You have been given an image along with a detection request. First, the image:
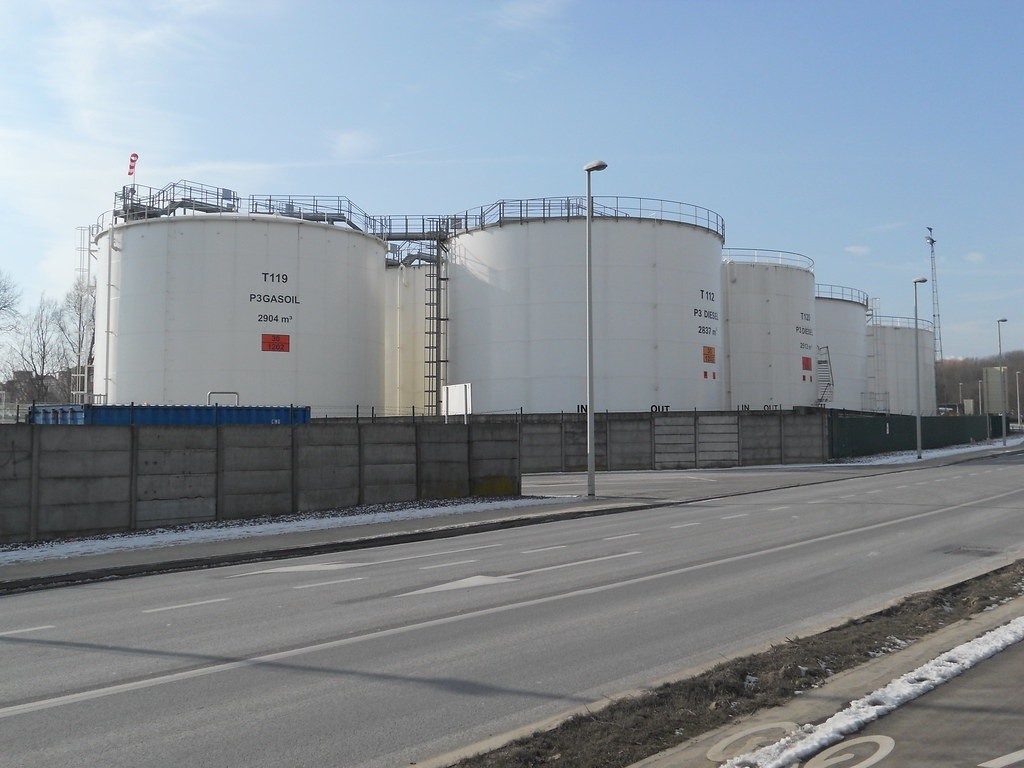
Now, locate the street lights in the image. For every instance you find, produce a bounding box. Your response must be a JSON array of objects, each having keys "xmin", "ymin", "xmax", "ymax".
[
  {"xmin": 979, "ymin": 380, "xmax": 982, "ymax": 416},
  {"xmin": 584, "ymin": 160, "xmax": 608, "ymax": 496},
  {"xmin": 1016, "ymin": 372, "xmax": 1021, "ymax": 425},
  {"xmin": 913, "ymin": 277, "xmax": 927, "ymax": 460},
  {"xmin": 997, "ymin": 318, "xmax": 1007, "ymax": 445},
  {"xmin": 959, "ymin": 383, "xmax": 963, "ymax": 404}
]
